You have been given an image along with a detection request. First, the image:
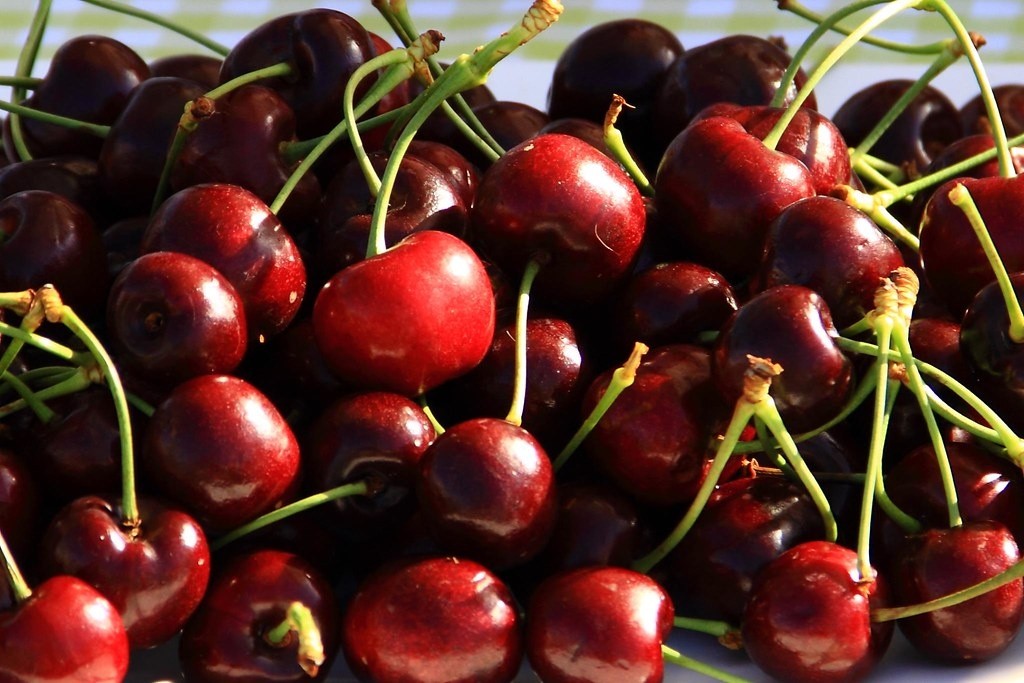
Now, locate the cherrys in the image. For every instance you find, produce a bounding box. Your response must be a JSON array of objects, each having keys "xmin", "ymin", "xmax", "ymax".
[{"xmin": 0, "ymin": 2, "xmax": 1024, "ymax": 683}]
